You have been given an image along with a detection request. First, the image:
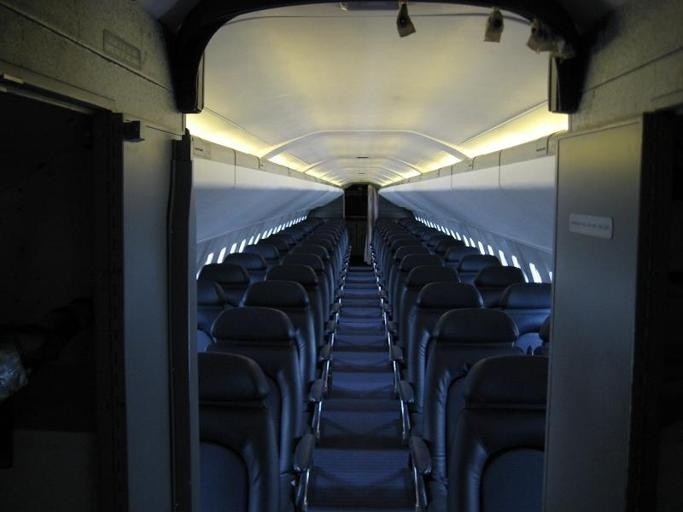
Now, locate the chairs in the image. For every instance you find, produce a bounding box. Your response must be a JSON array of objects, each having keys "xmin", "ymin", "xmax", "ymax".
[
  {"xmin": 194, "ymin": 215, "xmax": 348, "ymax": 512},
  {"xmin": 372, "ymin": 216, "xmax": 552, "ymax": 512}
]
